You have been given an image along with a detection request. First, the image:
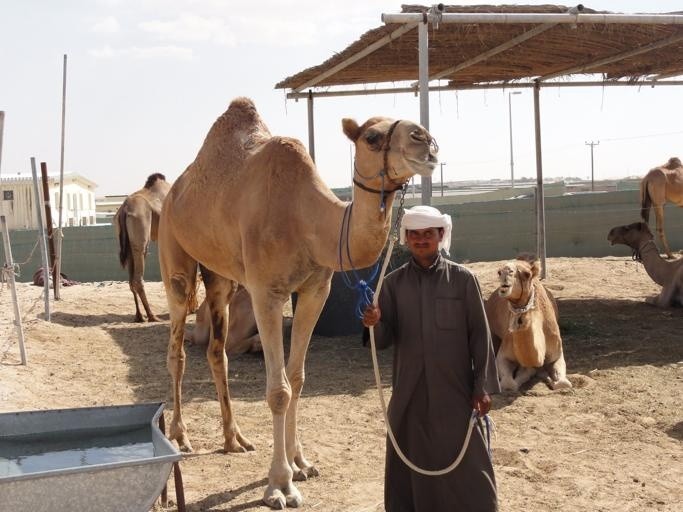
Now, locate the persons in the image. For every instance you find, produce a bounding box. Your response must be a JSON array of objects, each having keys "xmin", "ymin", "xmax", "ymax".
[{"xmin": 360, "ymin": 204, "xmax": 502, "ymax": 511}]
